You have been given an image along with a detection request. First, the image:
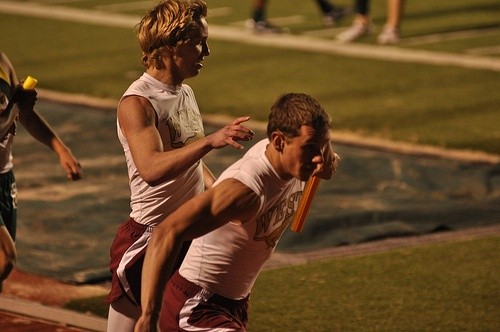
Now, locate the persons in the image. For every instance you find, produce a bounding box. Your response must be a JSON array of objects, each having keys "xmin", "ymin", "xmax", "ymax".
[
  {"xmin": 246, "ymin": 0, "xmax": 347, "ymax": 37},
  {"xmin": 337, "ymin": 0, "xmax": 406, "ymax": 44},
  {"xmin": 104, "ymin": 0, "xmax": 254, "ymax": 332},
  {"xmin": 0, "ymin": 49, "xmax": 84, "ymax": 293},
  {"xmin": 136, "ymin": 92, "xmax": 340, "ymax": 332}
]
